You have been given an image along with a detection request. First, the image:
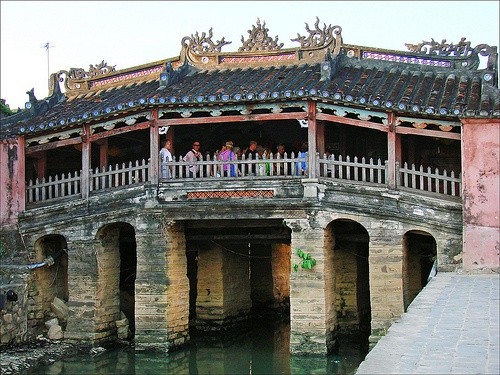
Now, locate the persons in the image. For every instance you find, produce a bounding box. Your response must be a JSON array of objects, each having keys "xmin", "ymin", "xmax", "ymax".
[
  {"xmin": 325, "ymin": 148, "xmax": 339, "ymax": 178},
  {"xmin": 159, "ymin": 139, "xmax": 174, "ymax": 180},
  {"xmin": 273, "ymin": 141, "xmax": 286, "ymax": 177},
  {"xmin": 241, "ymin": 141, "xmax": 276, "ymax": 176},
  {"xmin": 295, "ymin": 142, "xmax": 308, "ymax": 176},
  {"xmin": 207, "ymin": 140, "xmax": 242, "ymax": 177},
  {"xmin": 184, "ymin": 141, "xmax": 203, "ymax": 179}
]
